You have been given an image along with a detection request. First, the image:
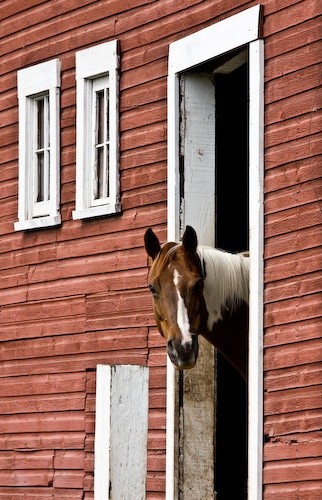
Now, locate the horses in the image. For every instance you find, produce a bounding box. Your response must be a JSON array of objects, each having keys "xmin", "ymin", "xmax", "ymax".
[{"xmin": 143, "ymin": 224, "xmax": 250, "ymax": 382}]
